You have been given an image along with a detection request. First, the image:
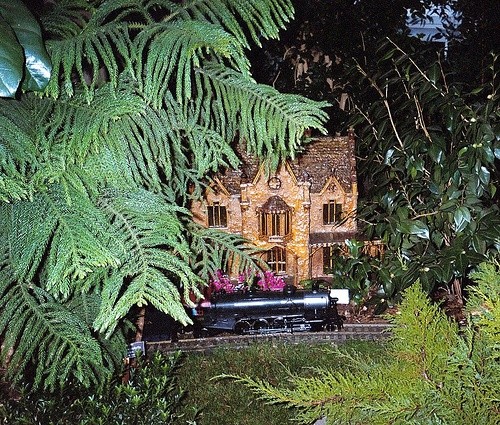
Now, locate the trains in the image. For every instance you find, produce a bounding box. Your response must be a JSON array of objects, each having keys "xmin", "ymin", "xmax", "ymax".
[{"xmin": 144, "ymin": 279, "xmax": 346, "ymax": 335}]
